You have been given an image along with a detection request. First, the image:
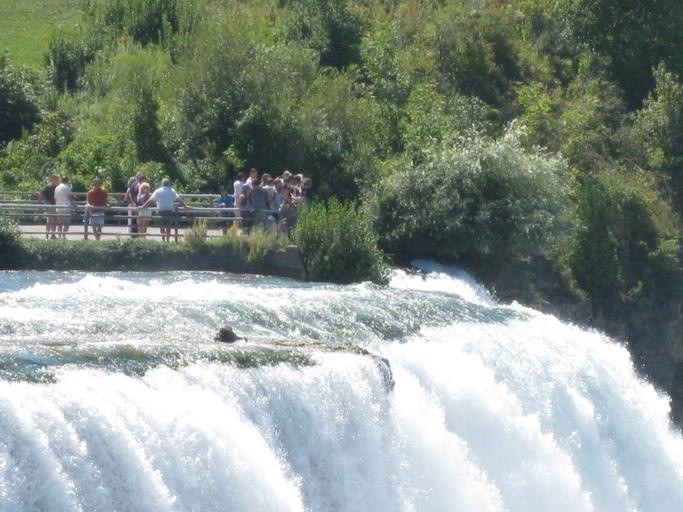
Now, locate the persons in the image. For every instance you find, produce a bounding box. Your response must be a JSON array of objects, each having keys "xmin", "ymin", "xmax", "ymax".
[
  {"xmin": 234, "ymin": 168, "xmax": 312, "ymax": 235},
  {"xmin": 40, "ymin": 176, "xmax": 58, "ymax": 239},
  {"xmin": 87, "ymin": 179, "xmax": 109, "ymax": 240},
  {"xmin": 217, "ymin": 187, "xmax": 235, "ymax": 235},
  {"xmin": 124, "ymin": 177, "xmax": 138, "ymax": 232},
  {"xmin": 138, "ymin": 180, "xmax": 189, "ymax": 242},
  {"xmin": 54, "ymin": 176, "xmax": 72, "ymax": 239},
  {"xmin": 129, "ymin": 172, "xmax": 146, "ymax": 238},
  {"xmin": 137, "ymin": 182, "xmax": 150, "ymax": 238}
]
